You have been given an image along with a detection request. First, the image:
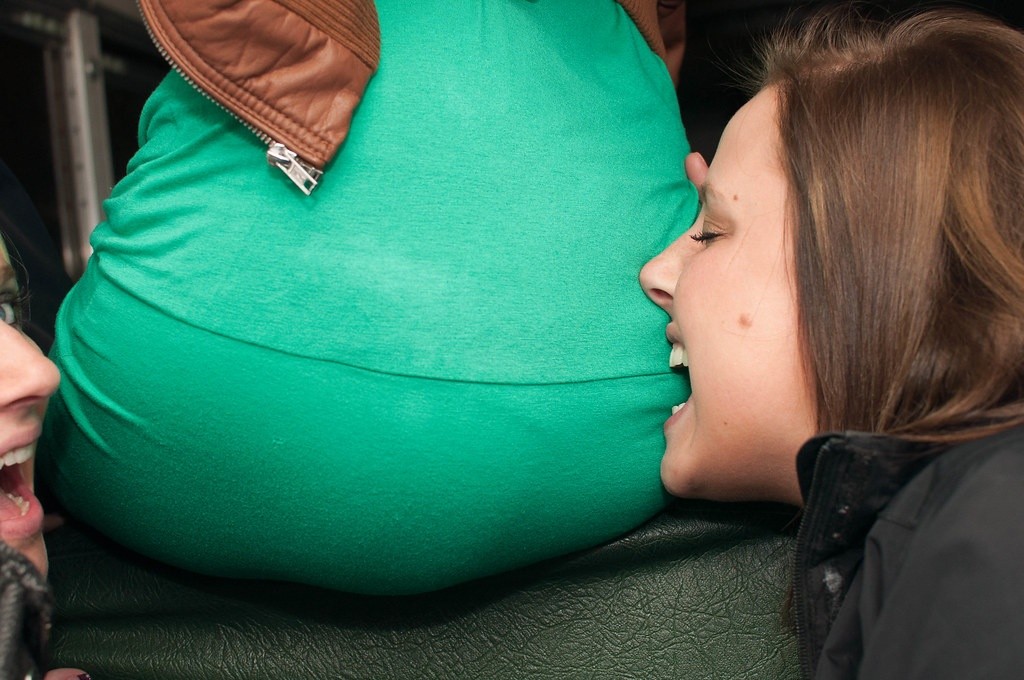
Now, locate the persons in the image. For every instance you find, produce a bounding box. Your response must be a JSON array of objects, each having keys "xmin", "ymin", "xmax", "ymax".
[
  {"xmin": 641, "ymin": 13, "xmax": 1023, "ymax": 679},
  {"xmin": 0, "ymin": 207, "xmax": 89, "ymax": 679}
]
